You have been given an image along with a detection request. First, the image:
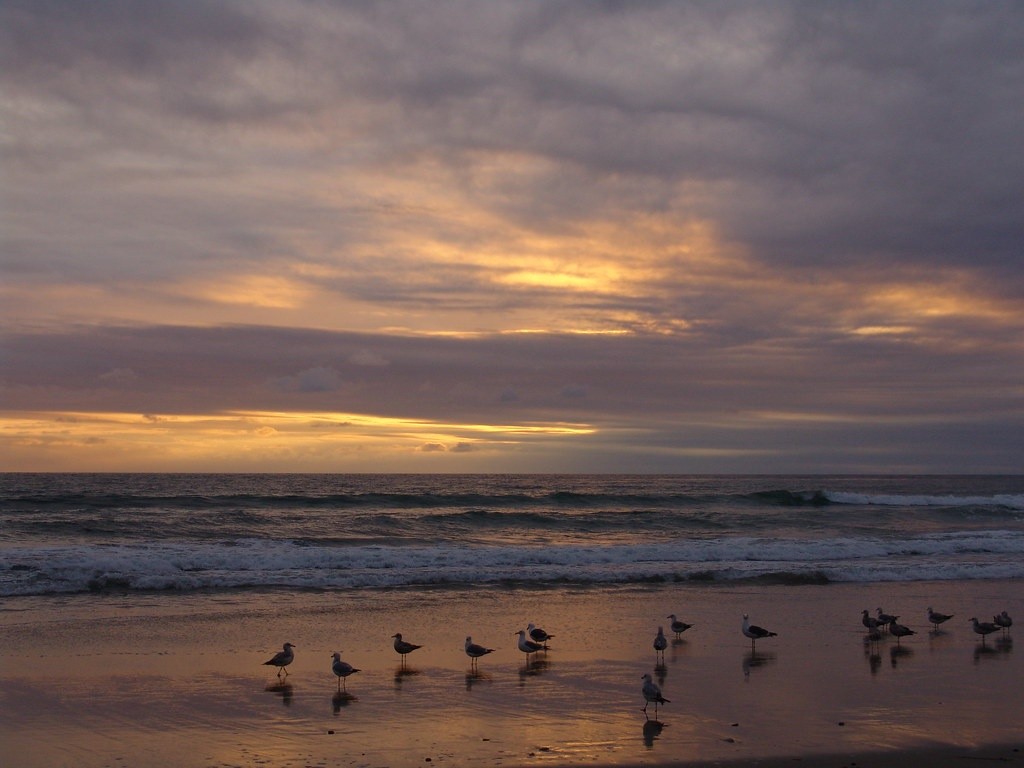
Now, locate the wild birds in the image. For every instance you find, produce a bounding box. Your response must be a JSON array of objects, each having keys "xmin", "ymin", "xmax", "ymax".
[
  {"xmin": 926, "ymin": 606, "xmax": 955, "ymax": 629},
  {"xmin": 261, "ymin": 643, "xmax": 297, "ymax": 677},
  {"xmin": 464, "ymin": 635, "xmax": 496, "ymax": 665},
  {"xmin": 860, "ymin": 607, "xmax": 918, "ymax": 647},
  {"xmin": 654, "ymin": 626, "xmax": 668, "ymax": 655},
  {"xmin": 391, "ymin": 632, "xmax": 424, "ymax": 660},
  {"xmin": 968, "ymin": 610, "xmax": 1014, "ymax": 642},
  {"xmin": 639, "ymin": 672, "xmax": 671, "ymax": 712},
  {"xmin": 667, "ymin": 614, "xmax": 695, "ymax": 638},
  {"xmin": 514, "ymin": 622, "xmax": 558, "ymax": 659},
  {"xmin": 741, "ymin": 613, "xmax": 778, "ymax": 648},
  {"xmin": 330, "ymin": 652, "xmax": 362, "ymax": 682}
]
